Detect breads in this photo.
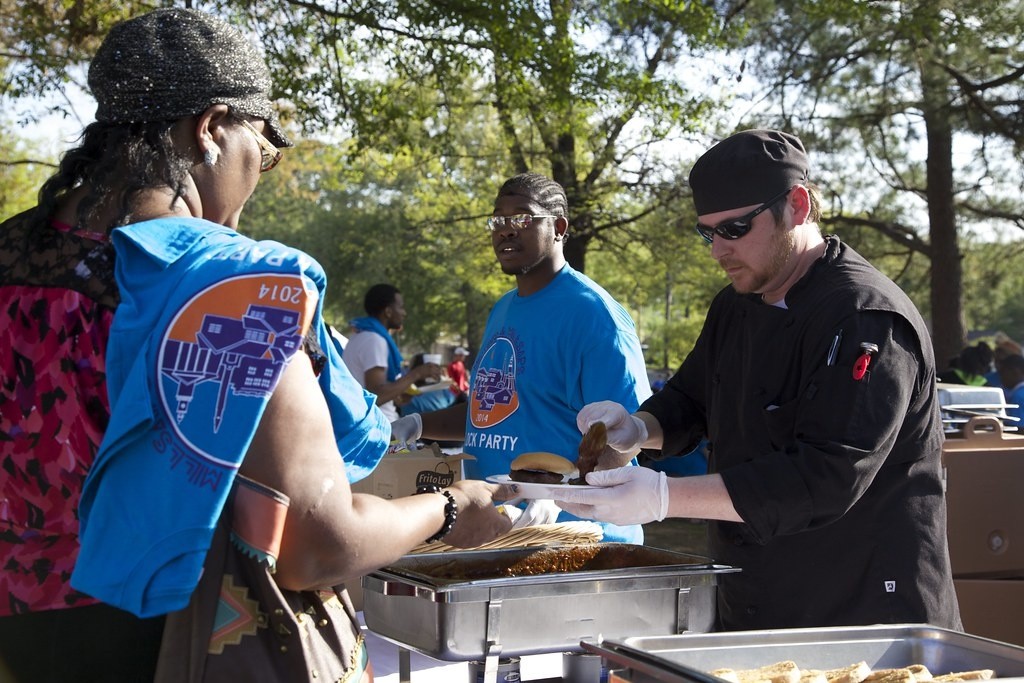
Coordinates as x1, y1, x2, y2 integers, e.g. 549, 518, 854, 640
708, 660, 998, 683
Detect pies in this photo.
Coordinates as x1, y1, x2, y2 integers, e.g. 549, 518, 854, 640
411, 520, 603, 553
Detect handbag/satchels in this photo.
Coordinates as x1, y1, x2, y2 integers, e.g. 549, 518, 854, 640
151, 471, 374, 683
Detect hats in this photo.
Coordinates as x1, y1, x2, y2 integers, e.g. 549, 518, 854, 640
455, 348, 469, 356
87, 7, 293, 148
688, 129, 810, 216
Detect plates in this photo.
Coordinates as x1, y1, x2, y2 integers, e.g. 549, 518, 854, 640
485, 474, 606, 499
418, 378, 454, 394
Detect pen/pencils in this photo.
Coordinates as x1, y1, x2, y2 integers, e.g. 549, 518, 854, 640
827, 329, 843, 366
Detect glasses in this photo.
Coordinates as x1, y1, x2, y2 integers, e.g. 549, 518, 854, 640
696, 187, 810, 243
195, 107, 283, 172
486, 215, 558, 231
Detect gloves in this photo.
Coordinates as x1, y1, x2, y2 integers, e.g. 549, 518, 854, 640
576, 400, 649, 453
554, 466, 669, 526
390, 412, 423, 446
503, 498, 562, 533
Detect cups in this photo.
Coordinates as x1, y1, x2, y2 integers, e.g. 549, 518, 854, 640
423, 354, 441, 382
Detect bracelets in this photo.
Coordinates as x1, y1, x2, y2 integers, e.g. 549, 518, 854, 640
410, 486, 458, 545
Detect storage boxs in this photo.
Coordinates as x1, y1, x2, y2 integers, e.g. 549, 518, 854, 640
345, 442, 477, 610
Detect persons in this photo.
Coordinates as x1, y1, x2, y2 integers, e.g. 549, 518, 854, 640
555, 129, 970, 636
446, 347, 470, 400
389, 173, 658, 548
0, 7, 520, 683
338, 284, 442, 425
937, 340, 1024, 434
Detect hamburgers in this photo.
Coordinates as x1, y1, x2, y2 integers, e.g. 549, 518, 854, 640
509, 453, 575, 484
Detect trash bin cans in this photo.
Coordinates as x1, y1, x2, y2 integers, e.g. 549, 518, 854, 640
937, 383, 1007, 433
